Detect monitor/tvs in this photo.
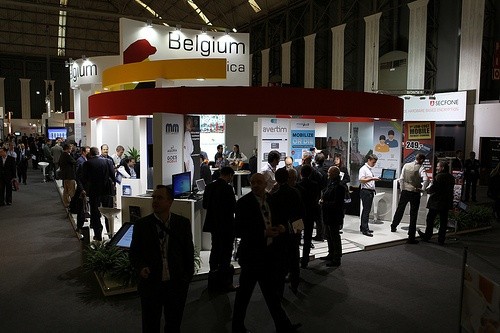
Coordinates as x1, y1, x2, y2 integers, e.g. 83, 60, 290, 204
382, 169, 396, 179
172, 171, 191, 197
47, 127, 68, 140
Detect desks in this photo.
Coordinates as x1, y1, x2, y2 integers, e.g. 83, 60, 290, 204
121, 193, 203, 256
233, 170, 251, 198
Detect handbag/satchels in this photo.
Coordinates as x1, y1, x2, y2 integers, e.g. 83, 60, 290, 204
69, 190, 82, 213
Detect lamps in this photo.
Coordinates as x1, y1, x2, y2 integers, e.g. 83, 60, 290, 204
147, 20, 207, 34
65, 54, 94, 67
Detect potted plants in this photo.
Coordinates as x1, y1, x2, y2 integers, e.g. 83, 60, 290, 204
81, 239, 131, 290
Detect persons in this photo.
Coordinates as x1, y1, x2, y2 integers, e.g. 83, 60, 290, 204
358, 155, 381, 237
0, 148, 17, 206
464, 152, 480, 202
201, 144, 351, 292
390, 153, 429, 245
59, 139, 140, 242
183, 117, 195, 194
232, 172, 302, 333
0, 133, 63, 185
452, 150, 465, 201
419, 161, 455, 243
129, 186, 194, 333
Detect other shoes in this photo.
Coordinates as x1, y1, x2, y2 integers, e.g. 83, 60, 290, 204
418, 230, 426, 241
326, 262, 340, 266
320, 256, 331, 260
366, 233, 373, 237
292, 322, 302, 332
93, 236, 102, 241
77, 233, 85, 240
312, 236, 324, 242
288, 285, 297, 293
368, 230, 373, 233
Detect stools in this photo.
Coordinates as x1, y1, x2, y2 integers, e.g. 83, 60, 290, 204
38, 162, 49, 183
98, 207, 120, 239
369, 192, 385, 224
